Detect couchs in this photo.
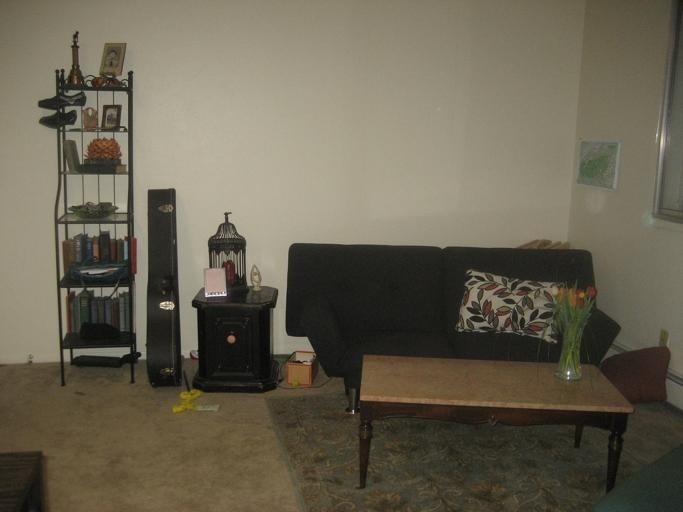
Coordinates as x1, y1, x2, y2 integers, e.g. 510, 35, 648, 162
283, 237, 621, 418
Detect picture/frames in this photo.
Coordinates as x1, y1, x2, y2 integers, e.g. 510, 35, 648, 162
97, 41, 127, 77
99, 103, 122, 132
573, 138, 622, 193
645, 0, 683, 228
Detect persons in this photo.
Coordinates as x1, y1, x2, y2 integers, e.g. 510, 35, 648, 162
105, 50, 118, 67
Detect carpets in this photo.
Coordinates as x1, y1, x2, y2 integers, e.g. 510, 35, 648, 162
259, 382, 682, 512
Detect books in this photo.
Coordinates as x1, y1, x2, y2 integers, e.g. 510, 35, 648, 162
63, 140, 80, 174
63, 232, 136, 333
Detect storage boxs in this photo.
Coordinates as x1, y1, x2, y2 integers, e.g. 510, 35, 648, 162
283, 349, 320, 387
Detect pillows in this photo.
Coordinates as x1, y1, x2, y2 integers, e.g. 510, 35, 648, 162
595, 343, 673, 405
454, 265, 574, 346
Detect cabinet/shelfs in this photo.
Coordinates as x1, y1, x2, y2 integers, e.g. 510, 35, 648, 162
185, 283, 284, 395
51, 66, 138, 389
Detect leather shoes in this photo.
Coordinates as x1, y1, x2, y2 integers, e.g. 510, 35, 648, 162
38, 91, 87, 109
38, 109, 77, 129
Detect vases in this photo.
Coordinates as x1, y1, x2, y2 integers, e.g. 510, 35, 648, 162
551, 307, 593, 382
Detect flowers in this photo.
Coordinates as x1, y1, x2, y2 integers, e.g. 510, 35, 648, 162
525, 276, 607, 385
